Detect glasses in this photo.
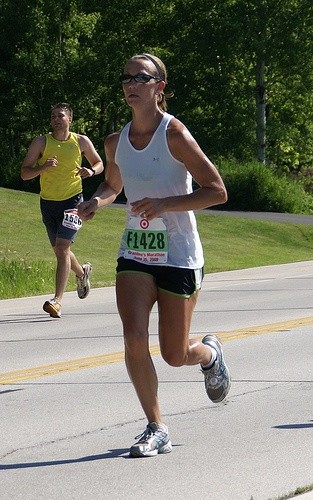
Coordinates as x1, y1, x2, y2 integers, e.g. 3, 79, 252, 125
119, 72, 161, 84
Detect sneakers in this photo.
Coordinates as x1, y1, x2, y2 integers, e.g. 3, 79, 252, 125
130, 422, 172, 457
76, 262, 92, 299
201, 334, 231, 403
43, 298, 62, 318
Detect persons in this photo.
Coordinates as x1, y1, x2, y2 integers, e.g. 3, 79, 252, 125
21, 102, 104, 318
76, 53, 233, 459
